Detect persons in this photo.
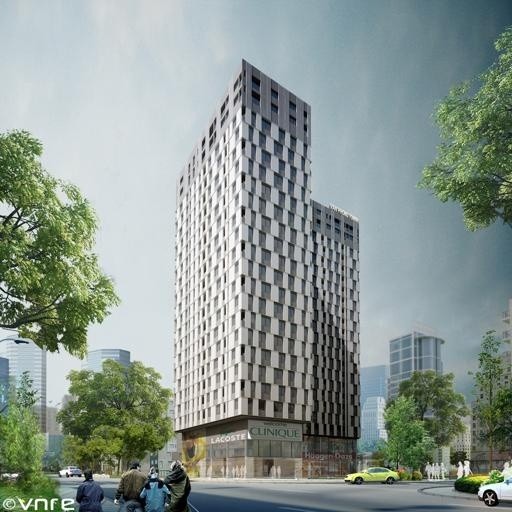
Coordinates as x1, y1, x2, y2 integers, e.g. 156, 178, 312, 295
114, 461, 147, 511
164, 459, 192, 512
140, 466, 171, 511
76, 469, 105, 511
